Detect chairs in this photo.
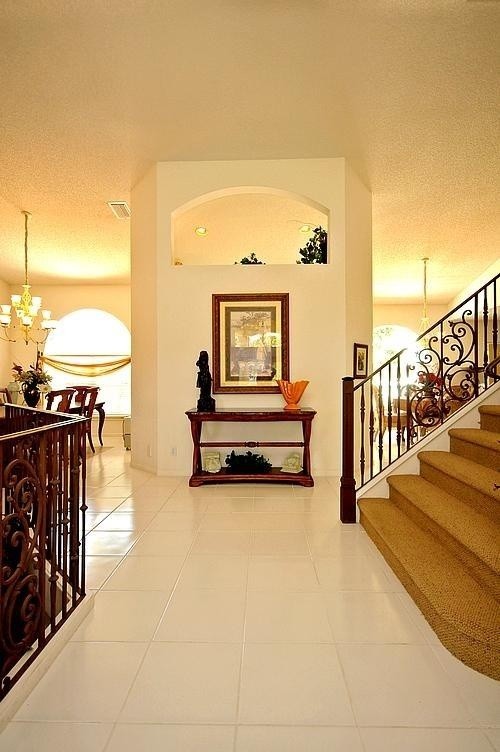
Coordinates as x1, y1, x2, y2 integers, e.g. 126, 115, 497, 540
0, 385, 99, 454
372, 385, 415, 447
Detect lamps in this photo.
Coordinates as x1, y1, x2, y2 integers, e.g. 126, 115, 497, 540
416, 257, 435, 347
0, 211, 59, 347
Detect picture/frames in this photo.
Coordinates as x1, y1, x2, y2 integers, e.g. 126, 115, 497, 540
354, 343, 368, 379
211, 292, 291, 395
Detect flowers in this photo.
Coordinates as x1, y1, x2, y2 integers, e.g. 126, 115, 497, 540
417, 370, 444, 389
8, 351, 53, 393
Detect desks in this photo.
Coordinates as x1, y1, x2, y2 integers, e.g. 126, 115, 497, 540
0, 401, 107, 447
392, 398, 430, 437
184, 407, 317, 488
